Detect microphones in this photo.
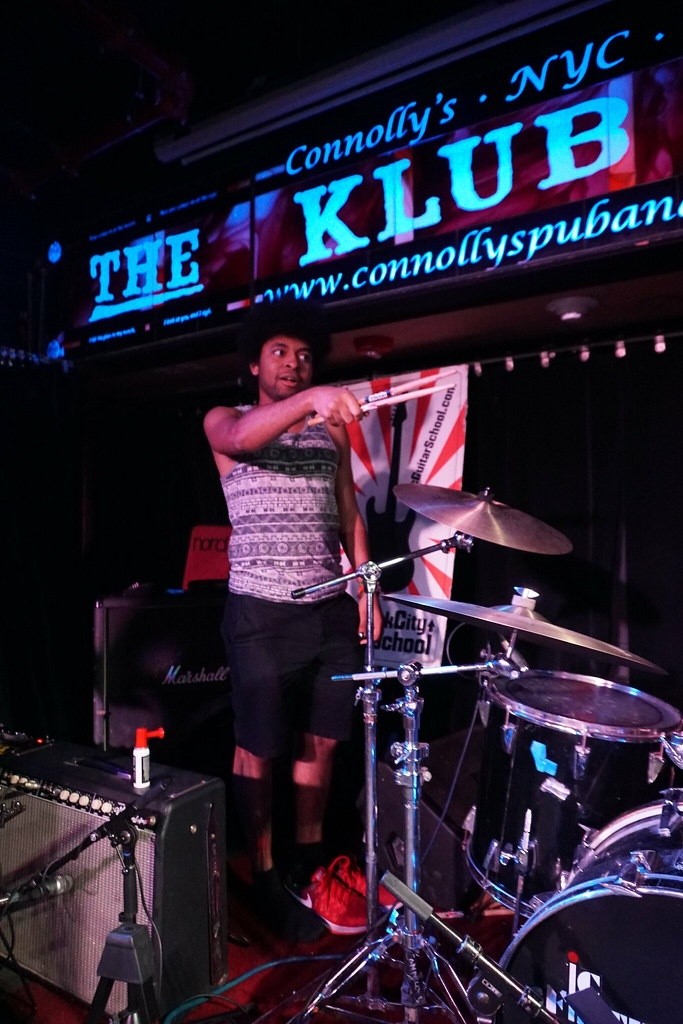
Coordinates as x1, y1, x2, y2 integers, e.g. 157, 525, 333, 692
0, 875, 72, 905
496, 628, 531, 671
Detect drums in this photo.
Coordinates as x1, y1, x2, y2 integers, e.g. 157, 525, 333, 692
461, 664, 683, 1024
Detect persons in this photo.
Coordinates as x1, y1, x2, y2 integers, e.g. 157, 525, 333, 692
202, 301, 387, 943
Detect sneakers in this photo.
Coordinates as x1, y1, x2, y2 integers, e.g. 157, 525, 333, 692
283, 864, 377, 935
327, 854, 403, 910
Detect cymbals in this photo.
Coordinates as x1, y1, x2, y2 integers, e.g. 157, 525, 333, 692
391, 481, 575, 558
380, 586, 666, 675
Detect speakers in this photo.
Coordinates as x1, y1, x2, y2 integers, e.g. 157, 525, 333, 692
0, 732, 228, 1024
355, 720, 514, 915
92, 598, 238, 760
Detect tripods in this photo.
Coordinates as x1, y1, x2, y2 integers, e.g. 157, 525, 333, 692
11, 779, 168, 1024
250, 542, 517, 1024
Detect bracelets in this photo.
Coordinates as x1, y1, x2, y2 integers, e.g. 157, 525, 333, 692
356, 580, 382, 599
